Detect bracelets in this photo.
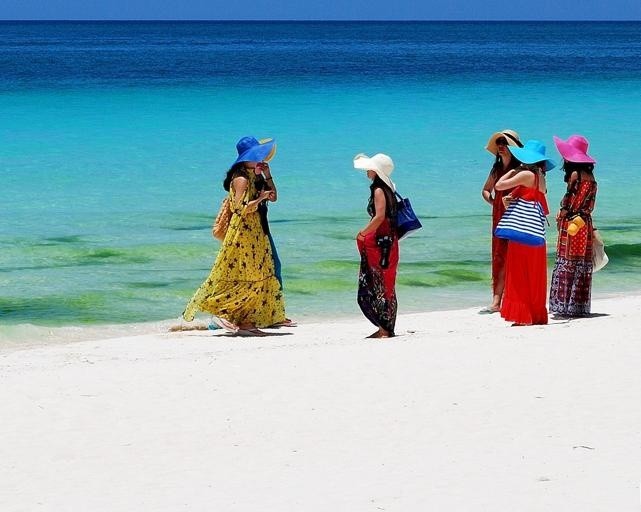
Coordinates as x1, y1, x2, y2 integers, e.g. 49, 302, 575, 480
359, 232, 365, 238
264, 177, 272, 181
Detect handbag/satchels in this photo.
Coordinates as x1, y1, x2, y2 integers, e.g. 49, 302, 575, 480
398, 197, 421, 240
494, 196, 545, 245
212, 197, 233, 241
591, 228, 609, 274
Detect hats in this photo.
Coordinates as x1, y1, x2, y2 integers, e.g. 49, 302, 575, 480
484, 129, 524, 157
553, 135, 597, 164
507, 140, 557, 171
229, 137, 276, 168
354, 153, 396, 194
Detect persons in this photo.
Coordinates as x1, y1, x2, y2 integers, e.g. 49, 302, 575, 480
477, 129, 525, 314
255, 138, 291, 325
179, 135, 287, 336
548, 134, 597, 319
494, 140, 556, 325
352, 153, 399, 337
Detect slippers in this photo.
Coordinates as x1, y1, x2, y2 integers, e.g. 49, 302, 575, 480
268, 318, 298, 327
213, 316, 236, 332
366, 331, 388, 337
238, 327, 268, 336
478, 307, 501, 314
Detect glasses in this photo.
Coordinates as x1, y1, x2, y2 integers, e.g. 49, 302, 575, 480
495, 139, 508, 146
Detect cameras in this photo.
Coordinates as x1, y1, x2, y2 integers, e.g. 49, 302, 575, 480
376, 233, 394, 269
